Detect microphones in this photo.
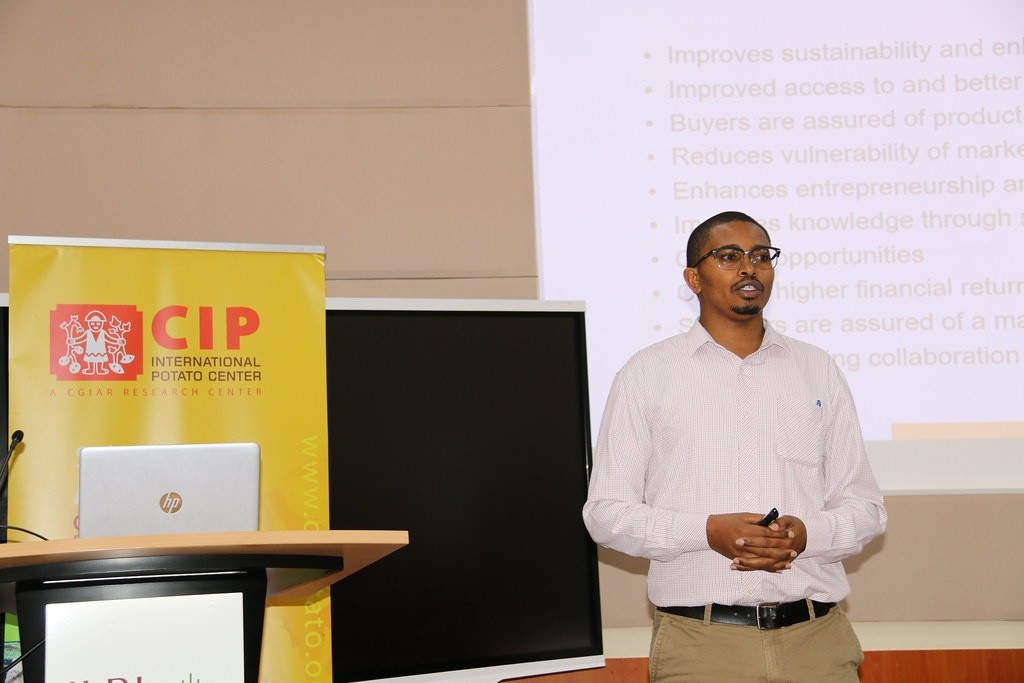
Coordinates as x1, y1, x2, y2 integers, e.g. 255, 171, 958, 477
1, 430, 23, 481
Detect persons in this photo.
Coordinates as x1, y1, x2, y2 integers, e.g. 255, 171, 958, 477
583, 210, 888, 683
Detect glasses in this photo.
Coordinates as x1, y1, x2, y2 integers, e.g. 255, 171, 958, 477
692, 246, 780, 271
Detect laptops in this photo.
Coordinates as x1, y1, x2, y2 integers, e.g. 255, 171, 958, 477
75, 443, 261, 539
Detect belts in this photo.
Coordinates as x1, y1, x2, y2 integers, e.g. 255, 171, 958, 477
656, 598, 837, 631
